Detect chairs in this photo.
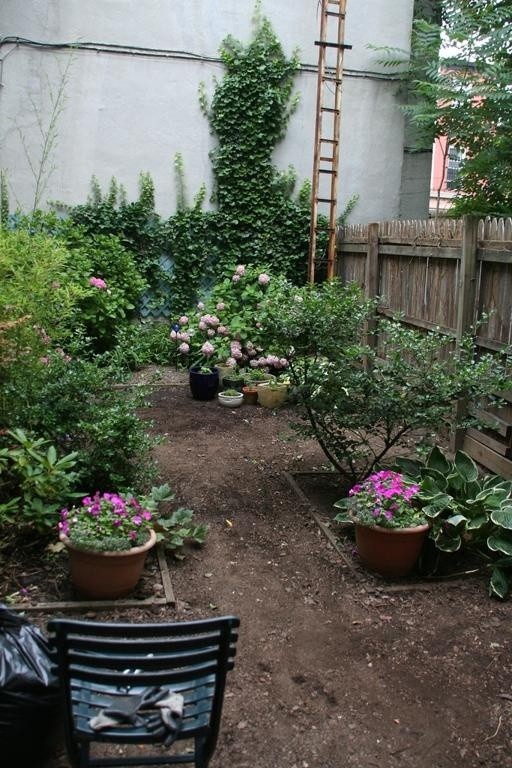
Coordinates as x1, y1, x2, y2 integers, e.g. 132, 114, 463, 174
45, 615, 241, 768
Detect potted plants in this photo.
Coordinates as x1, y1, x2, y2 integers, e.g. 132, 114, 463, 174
189, 366, 287, 408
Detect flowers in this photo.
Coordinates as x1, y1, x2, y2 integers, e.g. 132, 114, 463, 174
170, 263, 306, 374
57, 489, 153, 552
348, 470, 428, 528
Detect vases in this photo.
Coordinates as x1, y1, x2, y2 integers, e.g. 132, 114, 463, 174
59, 528, 157, 600
349, 509, 430, 579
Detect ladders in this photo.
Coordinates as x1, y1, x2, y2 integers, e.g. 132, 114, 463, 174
308, 0, 352, 285
